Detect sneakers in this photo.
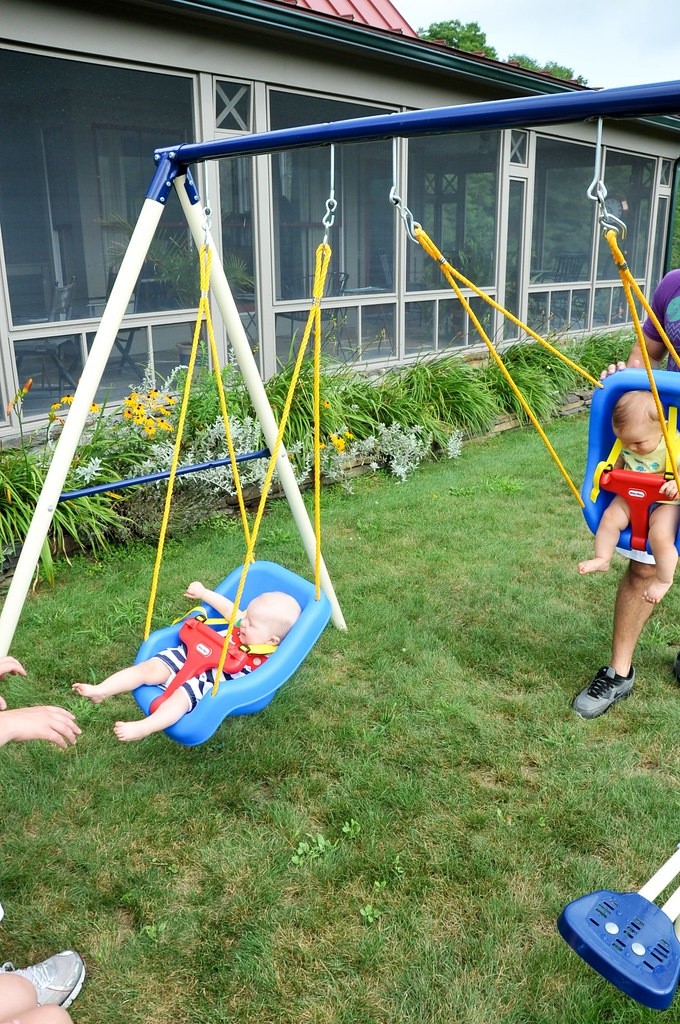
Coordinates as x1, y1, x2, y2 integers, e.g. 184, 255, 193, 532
2, 947, 88, 1013
572, 663, 637, 720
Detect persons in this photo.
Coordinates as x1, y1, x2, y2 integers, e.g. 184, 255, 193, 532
569, 268, 680, 721
578, 389, 680, 604
71, 581, 302, 741
0, 972, 74, 1024
0, 655, 88, 1010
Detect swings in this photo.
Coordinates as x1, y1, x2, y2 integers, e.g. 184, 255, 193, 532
132, 242, 332, 744
415, 229, 680, 555
